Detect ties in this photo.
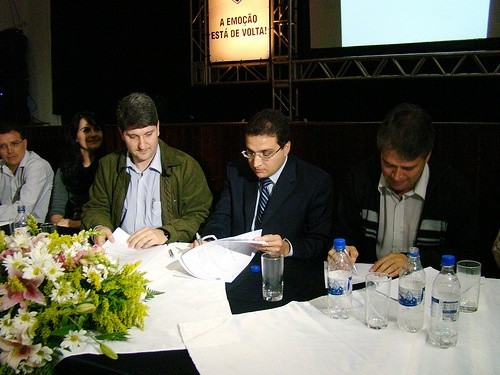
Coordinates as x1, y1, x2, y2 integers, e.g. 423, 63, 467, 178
254, 177, 272, 229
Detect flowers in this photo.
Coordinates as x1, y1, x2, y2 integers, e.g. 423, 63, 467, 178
0, 212, 164, 375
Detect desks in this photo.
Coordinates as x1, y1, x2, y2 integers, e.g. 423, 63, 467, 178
53, 251, 500, 375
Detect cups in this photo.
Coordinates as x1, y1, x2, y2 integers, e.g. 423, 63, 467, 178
365, 272, 392, 330
456, 260, 482, 314
9, 218, 15, 234
261, 251, 285, 302
40, 222, 57, 234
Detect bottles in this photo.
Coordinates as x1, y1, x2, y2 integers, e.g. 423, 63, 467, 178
426, 255, 461, 349
13, 204, 31, 236
396, 246, 426, 333
328, 238, 352, 320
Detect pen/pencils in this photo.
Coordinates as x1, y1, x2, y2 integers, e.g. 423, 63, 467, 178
196, 233, 202, 244
169, 249, 174, 256
344, 249, 360, 275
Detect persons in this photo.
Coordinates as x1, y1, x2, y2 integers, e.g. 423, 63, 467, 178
48, 110, 118, 228
81, 91, 212, 249
191, 110, 334, 261
326, 102, 481, 278
0, 123, 54, 236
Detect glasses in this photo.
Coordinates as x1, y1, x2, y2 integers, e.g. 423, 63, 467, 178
242, 146, 283, 161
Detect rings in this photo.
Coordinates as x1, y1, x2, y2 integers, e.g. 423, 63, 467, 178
265, 246, 268, 251
